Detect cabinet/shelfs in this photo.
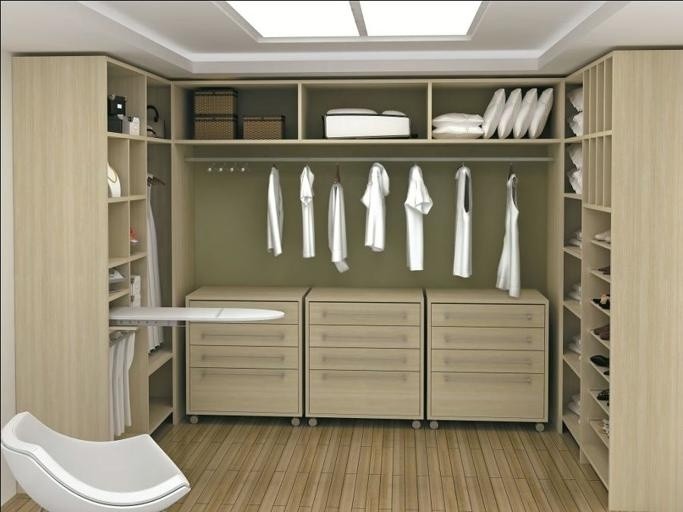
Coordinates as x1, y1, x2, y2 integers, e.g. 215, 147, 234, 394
172, 77, 565, 435
186, 287, 310, 425
11, 56, 172, 439
304, 287, 426, 429
426, 289, 550, 431
562, 50, 682, 511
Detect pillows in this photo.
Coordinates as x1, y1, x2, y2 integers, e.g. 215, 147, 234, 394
484, 88, 505, 140
432, 127, 483, 139
432, 113, 484, 126
529, 88, 554, 138
513, 88, 537, 138
499, 88, 521, 139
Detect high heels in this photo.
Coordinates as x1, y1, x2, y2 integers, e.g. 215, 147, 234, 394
593, 294, 610, 309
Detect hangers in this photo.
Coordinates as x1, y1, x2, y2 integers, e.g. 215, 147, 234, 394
148, 159, 518, 186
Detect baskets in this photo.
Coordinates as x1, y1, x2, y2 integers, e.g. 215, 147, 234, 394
193, 88, 284, 139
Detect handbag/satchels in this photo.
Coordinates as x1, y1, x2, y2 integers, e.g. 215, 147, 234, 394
147, 105, 165, 137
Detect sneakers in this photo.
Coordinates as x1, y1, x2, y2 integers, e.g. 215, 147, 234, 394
597, 389, 609, 406
598, 264, 610, 275
594, 324, 610, 340
595, 228, 610, 244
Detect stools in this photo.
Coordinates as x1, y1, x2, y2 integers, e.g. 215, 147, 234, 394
0, 412, 190, 510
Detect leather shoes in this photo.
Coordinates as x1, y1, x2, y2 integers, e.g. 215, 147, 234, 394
590, 354, 609, 375
